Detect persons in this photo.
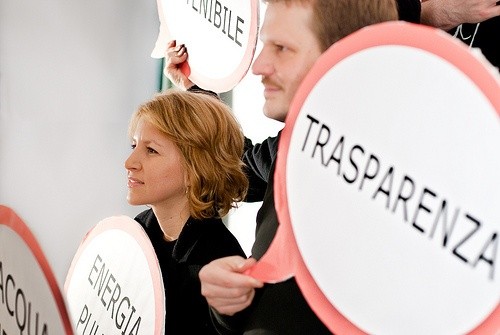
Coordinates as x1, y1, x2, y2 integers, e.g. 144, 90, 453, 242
163, 0, 398, 262
124, 88, 249, 335
419, 0, 500, 75
198, 255, 334, 335
